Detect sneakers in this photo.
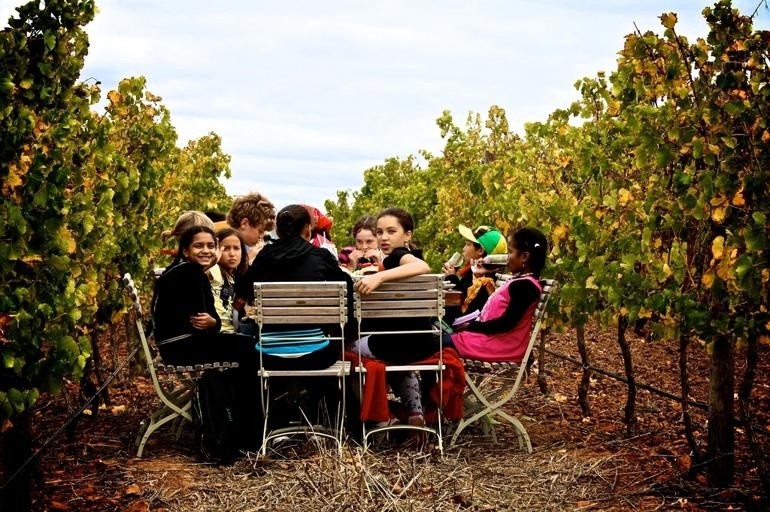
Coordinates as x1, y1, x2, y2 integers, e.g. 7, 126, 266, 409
376, 413, 427, 450
273, 434, 322, 450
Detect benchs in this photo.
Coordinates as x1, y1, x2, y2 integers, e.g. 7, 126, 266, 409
447, 272, 555, 455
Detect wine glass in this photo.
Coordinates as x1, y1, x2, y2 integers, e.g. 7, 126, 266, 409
470, 254, 508, 270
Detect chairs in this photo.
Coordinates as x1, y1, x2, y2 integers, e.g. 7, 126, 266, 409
254, 280, 352, 458
124, 269, 239, 457
353, 275, 448, 456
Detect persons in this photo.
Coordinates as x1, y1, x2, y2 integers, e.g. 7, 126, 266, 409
418, 224, 548, 451
149, 192, 432, 465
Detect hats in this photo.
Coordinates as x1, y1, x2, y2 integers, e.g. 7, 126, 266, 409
458, 224, 507, 254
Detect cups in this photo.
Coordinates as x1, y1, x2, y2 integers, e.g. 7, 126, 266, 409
446, 251, 464, 267
